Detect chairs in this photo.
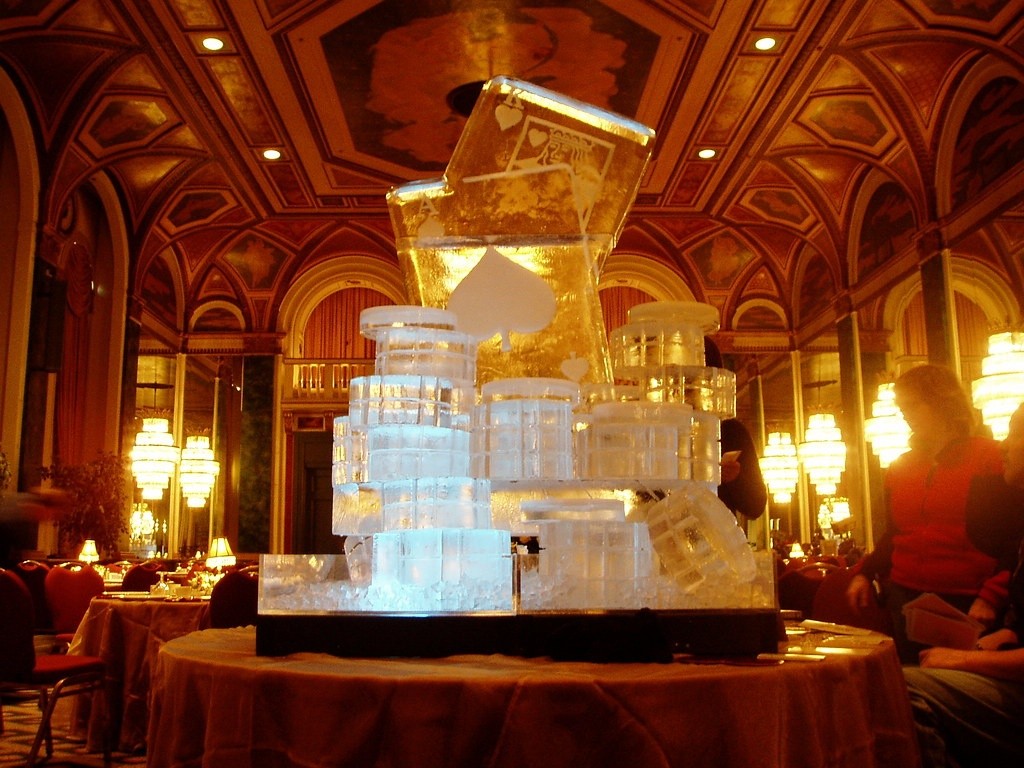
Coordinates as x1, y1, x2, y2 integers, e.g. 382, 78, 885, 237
771, 540, 892, 637
41, 560, 107, 655
10, 552, 259, 630
0, 568, 118, 768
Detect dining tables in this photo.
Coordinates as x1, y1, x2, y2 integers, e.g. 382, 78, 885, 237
144, 623, 924, 768
66, 591, 214, 758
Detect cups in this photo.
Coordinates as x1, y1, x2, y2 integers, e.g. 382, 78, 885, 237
110, 573, 122, 580
181, 568, 187, 572
176, 586, 191, 597
167, 584, 181, 597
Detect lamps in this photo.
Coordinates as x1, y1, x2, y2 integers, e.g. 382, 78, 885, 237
798, 402, 848, 496
205, 536, 236, 582
759, 419, 801, 504
865, 369, 917, 470
78, 540, 100, 567
179, 424, 220, 508
128, 406, 181, 500
972, 313, 1024, 442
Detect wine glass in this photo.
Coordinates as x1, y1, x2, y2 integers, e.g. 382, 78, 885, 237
153, 571, 170, 596
177, 563, 181, 572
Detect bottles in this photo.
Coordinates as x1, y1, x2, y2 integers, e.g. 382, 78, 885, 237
121, 565, 126, 579
106, 568, 110, 580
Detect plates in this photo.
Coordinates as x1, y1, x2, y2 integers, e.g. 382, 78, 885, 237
104, 591, 211, 601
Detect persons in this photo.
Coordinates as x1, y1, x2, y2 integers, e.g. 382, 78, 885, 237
511, 536, 540, 555
845, 363, 1018, 668
898, 401, 1024, 768
634, 334, 767, 521
